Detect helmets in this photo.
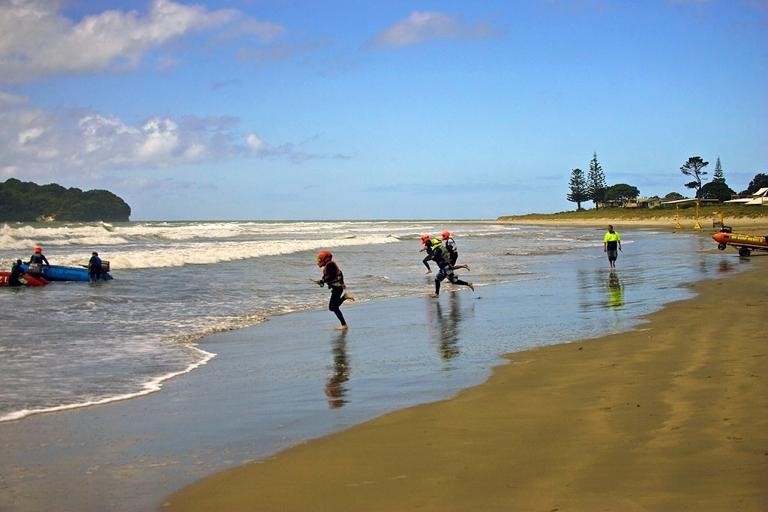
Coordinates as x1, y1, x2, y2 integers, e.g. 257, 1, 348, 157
35, 247, 42, 253
421, 234, 429, 243
316, 251, 333, 267
431, 238, 442, 251
442, 231, 450, 240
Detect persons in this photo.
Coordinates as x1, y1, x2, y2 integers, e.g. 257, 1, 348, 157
305, 250, 357, 333
418, 234, 436, 274
441, 231, 459, 283
430, 238, 475, 298
7, 259, 28, 288
21, 245, 50, 274
87, 251, 104, 286
602, 222, 624, 269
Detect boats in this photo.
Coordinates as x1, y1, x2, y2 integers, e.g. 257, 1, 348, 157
712, 225, 768, 250
12, 260, 114, 282
0, 272, 49, 287
